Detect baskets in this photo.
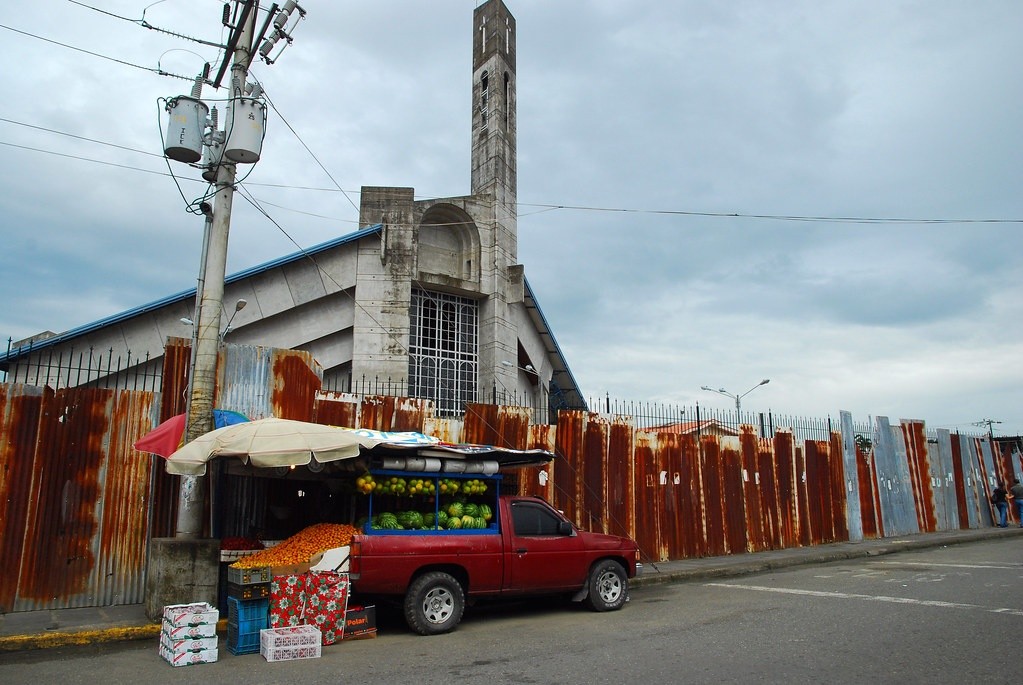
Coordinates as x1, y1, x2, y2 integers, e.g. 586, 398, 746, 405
259, 624, 321, 663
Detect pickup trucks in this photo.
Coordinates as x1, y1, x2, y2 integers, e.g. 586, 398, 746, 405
349, 494, 643, 635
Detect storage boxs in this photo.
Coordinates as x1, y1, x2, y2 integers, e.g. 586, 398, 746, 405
159, 601, 219, 667
220, 538, 377, 662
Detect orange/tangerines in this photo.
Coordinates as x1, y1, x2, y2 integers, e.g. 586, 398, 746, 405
357, 475, 488, 495
229, 522, 355, 567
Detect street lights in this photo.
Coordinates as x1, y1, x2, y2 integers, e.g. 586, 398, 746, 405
180, 299, 247, 337
701, 379, 770, 423
502, 361, 543, 393
174, 199, 231, 535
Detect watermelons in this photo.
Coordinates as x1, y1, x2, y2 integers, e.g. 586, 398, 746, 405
361, 495, 492, 535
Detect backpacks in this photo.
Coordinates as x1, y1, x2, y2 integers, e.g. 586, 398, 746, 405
990, 489, 998, 504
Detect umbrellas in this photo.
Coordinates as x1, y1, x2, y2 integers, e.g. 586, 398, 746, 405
131, 409, 253, 539
166, 414, 387, 477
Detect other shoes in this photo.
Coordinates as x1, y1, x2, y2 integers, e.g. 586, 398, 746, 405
1000, 524, 1007, 528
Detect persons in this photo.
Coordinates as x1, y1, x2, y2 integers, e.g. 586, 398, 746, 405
1009, 479, 1023, 528
993, 479, 1014, 528
